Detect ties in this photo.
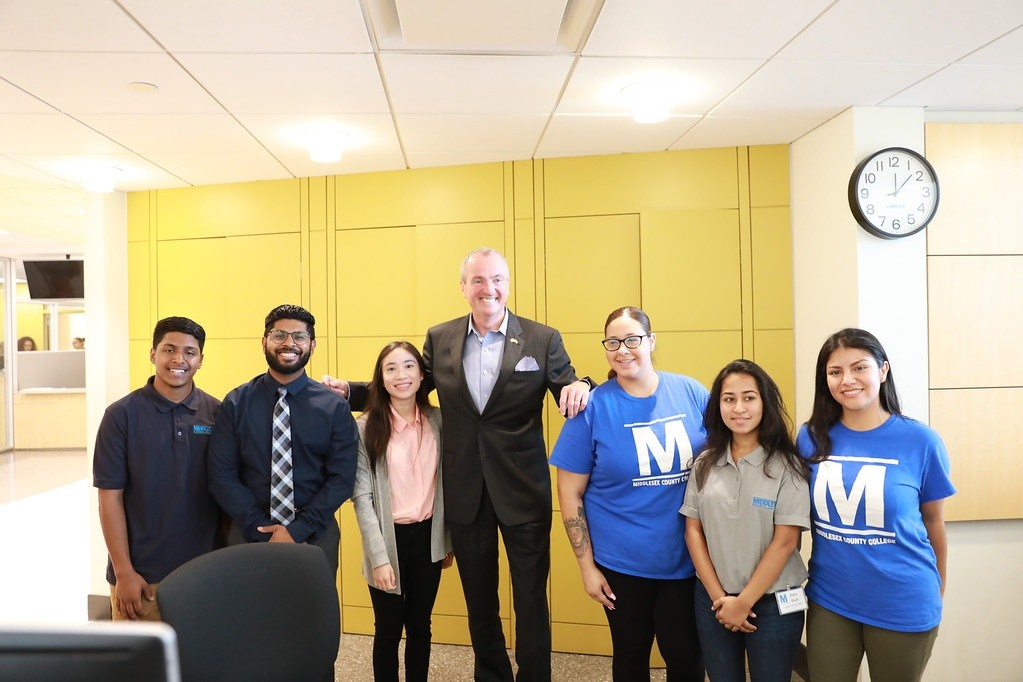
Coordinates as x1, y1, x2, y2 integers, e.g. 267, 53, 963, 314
270, 386, 295, 527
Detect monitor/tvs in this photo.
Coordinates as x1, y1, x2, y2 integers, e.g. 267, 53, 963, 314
23, 260, 84, 299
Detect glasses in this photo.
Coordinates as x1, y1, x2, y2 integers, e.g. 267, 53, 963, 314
265, 329, 311, 346
602, 334, 649, 352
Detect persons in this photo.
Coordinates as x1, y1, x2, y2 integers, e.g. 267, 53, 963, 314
797, 327, 957, 682
678, 359, 810, 682
206, 304, 359, 574
351, 341, 455, 682
547, 306, 711, 682
93, 316, 224, 625
18, 336, 36, 351
72, 337, 85, 349
321, 248, 599, 682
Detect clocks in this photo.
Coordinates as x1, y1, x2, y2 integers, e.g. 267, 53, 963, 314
847, 146, 941, 239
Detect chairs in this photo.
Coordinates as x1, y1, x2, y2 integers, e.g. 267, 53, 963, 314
156, 542, 341, 682
0, 622, 183, 682
